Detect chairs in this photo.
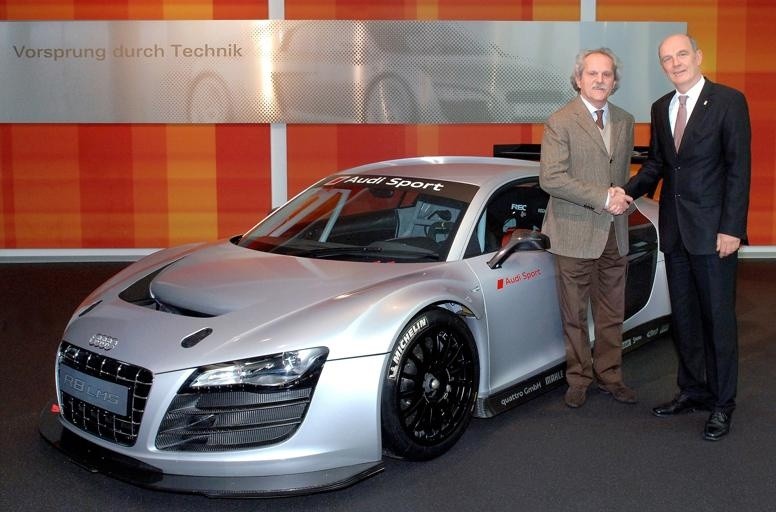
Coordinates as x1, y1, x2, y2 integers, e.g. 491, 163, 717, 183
409, 187, 543, 250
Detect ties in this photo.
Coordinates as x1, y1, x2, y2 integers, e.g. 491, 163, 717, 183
595, 109, 605, 130
672, 95, 690, 153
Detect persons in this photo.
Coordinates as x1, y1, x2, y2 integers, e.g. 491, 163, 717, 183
607, 34, 752, 440
540, 46, 640, 406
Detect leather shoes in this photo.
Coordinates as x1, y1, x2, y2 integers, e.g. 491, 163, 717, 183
597, 377, 641, 404
565, 382, 588, 409
650, 397, 695, 419
703, 408, 731, 442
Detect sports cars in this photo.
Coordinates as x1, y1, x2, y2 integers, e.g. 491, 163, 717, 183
170, 20, 578, 124
34, 141, 677, 498
